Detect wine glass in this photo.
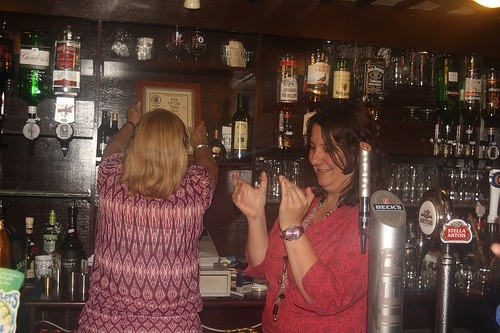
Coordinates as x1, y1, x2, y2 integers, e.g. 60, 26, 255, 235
167, 31, 187, 64
187, 32, 208, 64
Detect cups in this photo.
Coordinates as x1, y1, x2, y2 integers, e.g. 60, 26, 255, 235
34, 256, 86, 302
405, 266, 439, 291
455, 266, 491, 296
442, 167, 488, 205
254, 158, 303, 200
383, 162, 438, 207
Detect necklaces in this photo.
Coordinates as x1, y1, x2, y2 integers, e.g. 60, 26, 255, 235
303, 196, 342, 229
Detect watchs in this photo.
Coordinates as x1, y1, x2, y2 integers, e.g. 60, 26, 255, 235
280, 224, 304, 240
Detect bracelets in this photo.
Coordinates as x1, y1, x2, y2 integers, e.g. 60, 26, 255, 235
194, 144, 211, 150
123, 121, 136, 128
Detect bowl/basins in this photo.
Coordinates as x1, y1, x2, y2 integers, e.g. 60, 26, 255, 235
226, 170, 252, 193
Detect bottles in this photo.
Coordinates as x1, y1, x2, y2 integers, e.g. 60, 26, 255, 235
438, 54, 500, 158
96, 110, 119, 167
17, 217, 41, 286
219, 39, 249, 69
43, 210, 63, 278
111, 35, 155, 62
276, 42, 392, 158
20, 29, 50, 153
62, 207, 88, 280
53, 30, 80, 156
0, 220, 11, 268
208, 126, 226, 161
231, 94, 250, 162
390, 48, 438, 86
0, 20, 15, 135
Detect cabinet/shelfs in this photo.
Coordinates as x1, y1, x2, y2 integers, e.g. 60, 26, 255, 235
0, 10, 500, 268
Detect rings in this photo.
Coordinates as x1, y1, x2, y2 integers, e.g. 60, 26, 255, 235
205, 132, 209, 136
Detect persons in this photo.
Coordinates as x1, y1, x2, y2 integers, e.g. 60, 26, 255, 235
232, 101, 376, 333
78, 102, 218, 333
